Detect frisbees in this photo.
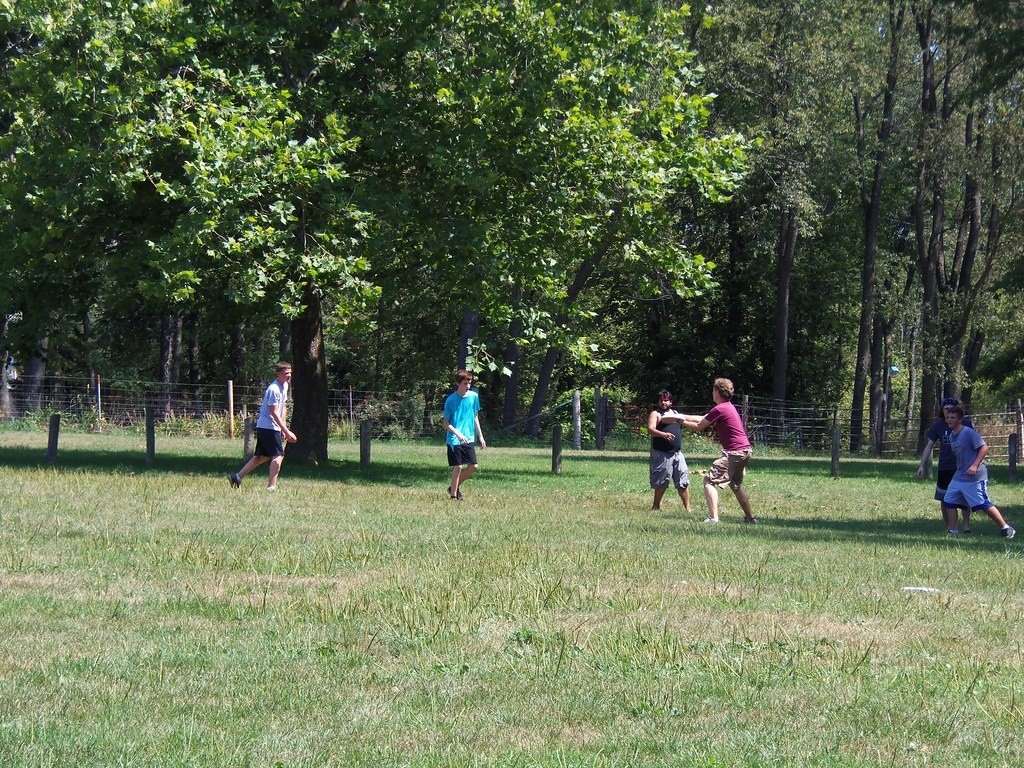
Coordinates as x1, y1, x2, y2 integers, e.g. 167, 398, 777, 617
661, 416, 686, 423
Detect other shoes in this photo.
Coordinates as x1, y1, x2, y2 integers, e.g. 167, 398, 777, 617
703, 516, 716, 523
965, 529, 971, 533
744, 516, 757, 523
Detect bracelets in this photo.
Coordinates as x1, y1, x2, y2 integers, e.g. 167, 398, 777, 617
676, 419, 681, 423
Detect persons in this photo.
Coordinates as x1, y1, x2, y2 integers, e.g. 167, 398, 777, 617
664, 378, 757, 526
647, 391, 701, 514
918, 399, 976, 535
5, 356, 17, 393
443, 370, 486, 501
941, 406, 1016, 540
228, 362, 297, 493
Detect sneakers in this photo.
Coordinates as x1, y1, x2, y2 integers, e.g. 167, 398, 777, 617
228, 475, 241, 487
447, 486, 463, 501
1000, 525, 1015, 539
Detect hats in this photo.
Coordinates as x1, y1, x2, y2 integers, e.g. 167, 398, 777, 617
939, 399, 958, 417
